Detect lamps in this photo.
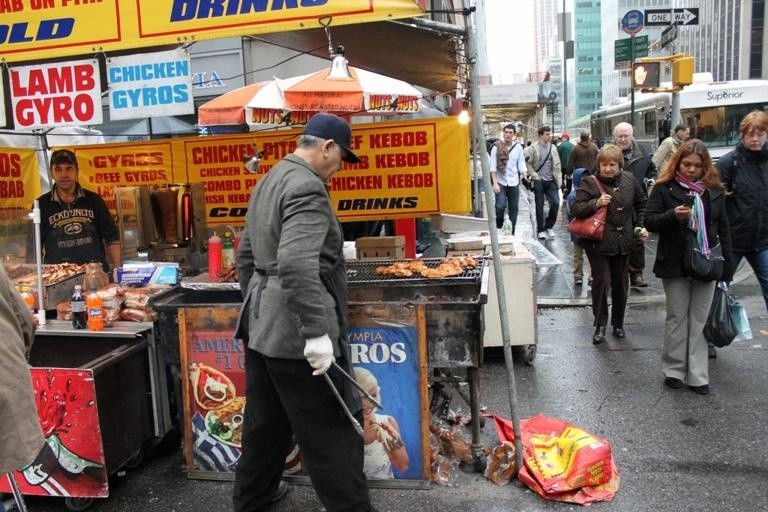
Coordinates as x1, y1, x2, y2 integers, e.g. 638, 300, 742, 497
456, 84, 471, 126
323, 20, 355, 83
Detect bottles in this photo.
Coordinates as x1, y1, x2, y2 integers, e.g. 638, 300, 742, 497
113, 268, 118, 282
71, 286, 87, 329
208, 231, 222, 280
90, 275, 97, 288
22, 287, 35, 315
222, 232, 236, 270
108, 264, 113, 283
14, 286, 22, 297
16, 433, 108, 498
117, 267, 123, 282
137, 247, 149, 261
87, 288, 104, 331
234, 228, 244, 257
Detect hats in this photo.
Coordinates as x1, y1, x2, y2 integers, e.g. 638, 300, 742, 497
51, 150, 78, 164
580, 127, 589, 139
299, 113, 362, 164
559, 132, 569, 140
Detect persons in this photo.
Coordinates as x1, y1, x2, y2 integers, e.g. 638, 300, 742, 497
351, 367, 410, 482
524, 126, 563, 240
557, 122, 692, 344
25, 150, 122, 283
232, 112, 378, 512
489, 123, 531, 235
644, 138, 733, 394
708, 110, 768, 359
0, 262, 45, 482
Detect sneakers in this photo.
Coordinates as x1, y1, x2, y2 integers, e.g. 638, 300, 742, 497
630, 272, 648, 287
538, 228, 546, 239
233, 479, 290, 512
545, 224, 555, 238
574, 276, 583, 284
707, 344, 717, 358
563, 188, 568, 200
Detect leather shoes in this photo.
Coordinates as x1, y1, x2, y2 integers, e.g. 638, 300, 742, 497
613, 320, 625, 338
665, 376, 682, 389
593, 326, 606, 344
689, 383, 709, 395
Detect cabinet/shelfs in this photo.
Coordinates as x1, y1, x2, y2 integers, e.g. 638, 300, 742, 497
0, 321, 170, 508
445, 230, 540, 364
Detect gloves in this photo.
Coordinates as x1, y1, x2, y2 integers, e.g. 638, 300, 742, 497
304, 333, 336, 375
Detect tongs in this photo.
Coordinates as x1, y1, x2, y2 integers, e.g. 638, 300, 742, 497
324, 363, 383, 437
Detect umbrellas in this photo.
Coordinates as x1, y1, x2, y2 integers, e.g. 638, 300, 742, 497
197, 64, 423, 131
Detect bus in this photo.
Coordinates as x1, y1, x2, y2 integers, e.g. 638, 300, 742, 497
586, 79, 768, 160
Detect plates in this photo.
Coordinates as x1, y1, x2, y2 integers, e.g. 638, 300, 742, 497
195, 364, 235, 410
204, 396, 248, 448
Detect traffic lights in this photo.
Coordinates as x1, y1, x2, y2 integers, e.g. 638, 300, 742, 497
632, 62, 660, 87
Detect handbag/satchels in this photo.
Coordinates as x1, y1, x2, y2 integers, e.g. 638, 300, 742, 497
522, 174, 535, 192
683, 232, 725, 281
567, 205, 608, 240
727, 293, 755, 343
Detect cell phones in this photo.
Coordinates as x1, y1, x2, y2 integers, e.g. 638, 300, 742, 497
682, 193, 696, 210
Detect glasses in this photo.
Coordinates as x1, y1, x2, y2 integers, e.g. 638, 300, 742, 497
504, 131, 514, 134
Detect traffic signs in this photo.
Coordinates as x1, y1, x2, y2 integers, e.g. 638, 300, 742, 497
634, 35, 649, 58
644, 10, 671, 26
614, 38, 631, 71
673, 8, 700, 26
660, 22, 677, 49
